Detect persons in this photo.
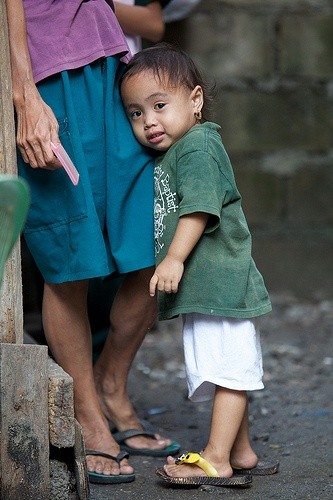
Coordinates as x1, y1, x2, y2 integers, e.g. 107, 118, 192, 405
115, 0, 203, 76
6, 0, 182, 483
120, 43, 280, 486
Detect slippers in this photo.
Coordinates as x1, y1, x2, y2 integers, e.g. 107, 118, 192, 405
156, 451, 252, 485
85, 440, 136, 482
230, 449, 279, 476
112, 416, 181, 457
